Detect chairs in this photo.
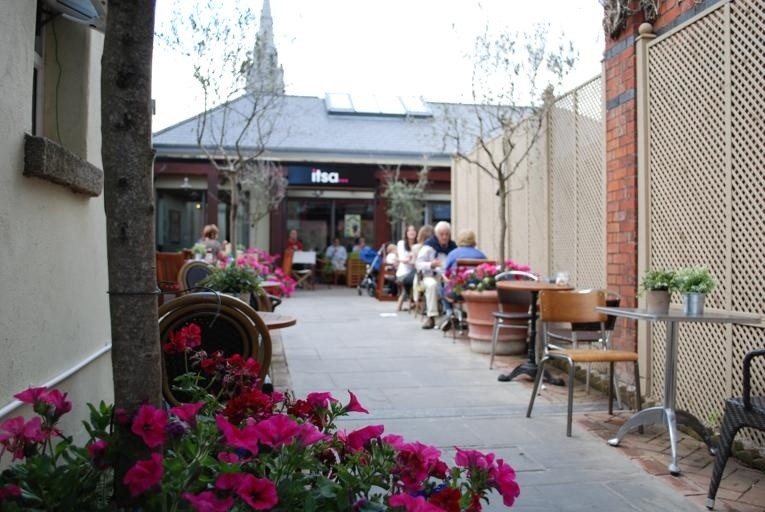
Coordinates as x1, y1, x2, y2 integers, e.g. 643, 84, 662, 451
178, 260, 214, 293
706, 349, 765, 511
158, 293, 272, 407
537, 289, 623, 409
445, 260, 495, 344
489, 270, 538, 369
283, 250, 426, 319
157, 250, 185, 304
184, 248, 213, 260
526, 291, 644, 436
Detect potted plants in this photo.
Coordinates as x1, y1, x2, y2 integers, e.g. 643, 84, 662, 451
670, 266, 714, 314
636, 270, 676, 313
194, 263, 265, 306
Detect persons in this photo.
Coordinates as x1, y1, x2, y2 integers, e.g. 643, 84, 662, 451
284, 228, 304, 274
193, 224, 232, 259
325, 237, 348, 269
351, 221, 488, 331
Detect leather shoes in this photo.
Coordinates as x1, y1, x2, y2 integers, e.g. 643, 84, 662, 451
421, 317, 434, 329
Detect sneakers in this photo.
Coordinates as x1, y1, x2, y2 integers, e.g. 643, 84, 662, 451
441, 316, 455, 331
